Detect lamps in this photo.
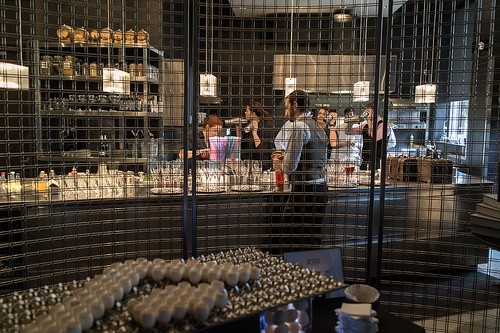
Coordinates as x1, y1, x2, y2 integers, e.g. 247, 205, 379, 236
285, 0, 296, 98
414, 0, 438, 103
0, 0, 30, 90
102, 0, 130, 95
353, 0, 370, 102
333, 0, 355, 24
200, 0, 217, 98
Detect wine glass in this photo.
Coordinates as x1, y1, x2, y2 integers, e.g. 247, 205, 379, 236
324, 158, 390, 187
146, 160, 264, 194
127, 126, 155, 140
68, 94, 121, 112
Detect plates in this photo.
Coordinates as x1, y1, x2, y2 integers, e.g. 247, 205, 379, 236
61, 149, 91, 158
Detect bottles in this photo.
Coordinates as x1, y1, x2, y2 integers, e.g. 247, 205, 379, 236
360, 111, 369, 121
1, 170, 144, 197
275, 155, 284, 192
121, 95, 164, 112
103, 134, 109, 157
98, 131, 105, 157
410, 133, 414, 146
244, 121, 259, 133
323, 115, 334, 123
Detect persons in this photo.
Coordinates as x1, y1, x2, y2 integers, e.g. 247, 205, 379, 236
179, 114, 223, 160
271, 90, 330, 244
316, 101, 397, 171
235, 101, 274, 170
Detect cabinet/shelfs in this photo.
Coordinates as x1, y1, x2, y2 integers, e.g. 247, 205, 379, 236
30, 40, 164, 173
387, 99, 430, 158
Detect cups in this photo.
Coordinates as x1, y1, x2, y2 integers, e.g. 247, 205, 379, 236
224, 118, 248, 124
344, 117, 359, 123
